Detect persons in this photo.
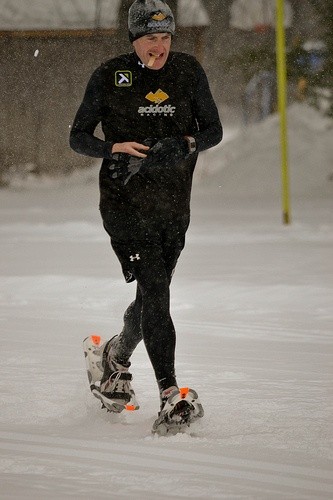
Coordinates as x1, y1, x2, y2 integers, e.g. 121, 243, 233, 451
69, 0, 224, 436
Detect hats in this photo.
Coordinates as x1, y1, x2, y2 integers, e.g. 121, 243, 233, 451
127, 0, 175, 44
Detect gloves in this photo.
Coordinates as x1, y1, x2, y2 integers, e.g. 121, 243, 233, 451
142, 138, 187, 175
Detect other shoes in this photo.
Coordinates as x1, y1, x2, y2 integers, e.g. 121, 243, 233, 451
99, 333, 131, 414
157, 392, 198, 425
